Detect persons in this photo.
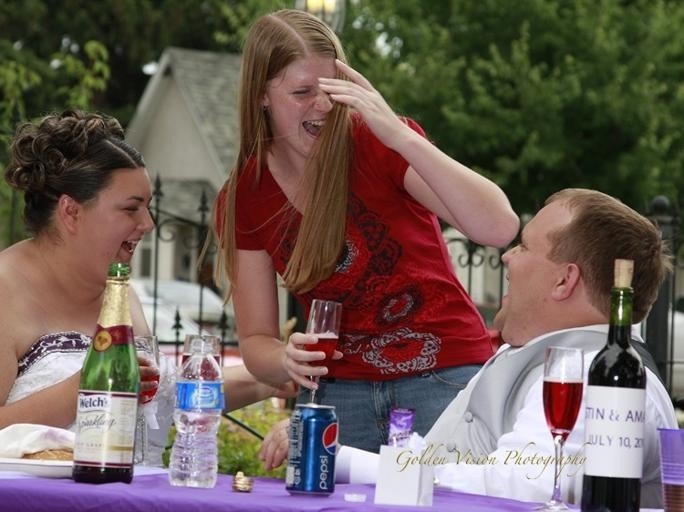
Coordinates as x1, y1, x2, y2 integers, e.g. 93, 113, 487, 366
211, 7, 522, 453
258, 187, 680, 506
0, 109, 300, 461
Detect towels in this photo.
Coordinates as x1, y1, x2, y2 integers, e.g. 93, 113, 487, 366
0, 423, 76, 459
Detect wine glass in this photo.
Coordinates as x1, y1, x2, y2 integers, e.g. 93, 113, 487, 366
135, 331, 160, 470
300, 298, 344, 407
537, 344, 586, 512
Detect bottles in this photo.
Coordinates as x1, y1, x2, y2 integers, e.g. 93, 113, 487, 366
576, 258, 649, 512
165, 337, 225, 490
70, 260, 141, 485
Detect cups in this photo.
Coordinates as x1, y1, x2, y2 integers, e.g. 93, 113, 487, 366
655, 426, 684, 512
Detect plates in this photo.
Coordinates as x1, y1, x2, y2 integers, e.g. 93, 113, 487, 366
0, 457, 75, 479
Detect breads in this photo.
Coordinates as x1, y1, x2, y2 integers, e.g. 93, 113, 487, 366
23, 451, 74, 460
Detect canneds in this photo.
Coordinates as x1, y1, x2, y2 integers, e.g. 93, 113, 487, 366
280, 402, 339, 496
